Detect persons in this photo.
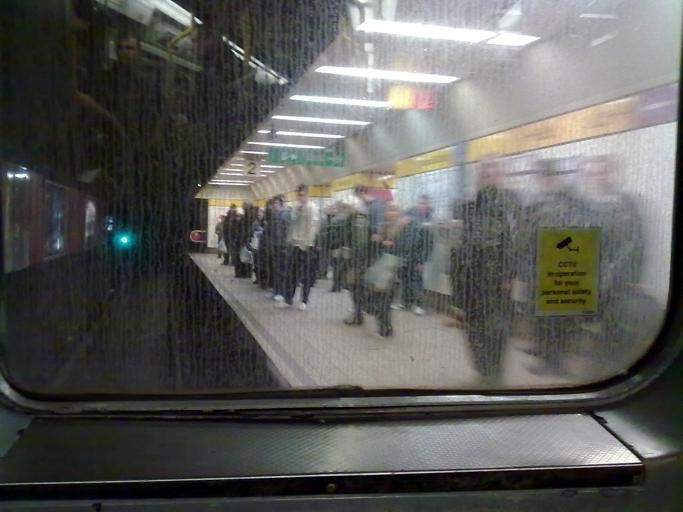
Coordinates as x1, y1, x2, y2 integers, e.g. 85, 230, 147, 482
214, 184, 434, 338
0, 0, 161, 138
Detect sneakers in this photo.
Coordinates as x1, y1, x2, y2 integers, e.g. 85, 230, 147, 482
265, 291, 307, 311
413, 304, 425, 315
390, 304, 406, 310
